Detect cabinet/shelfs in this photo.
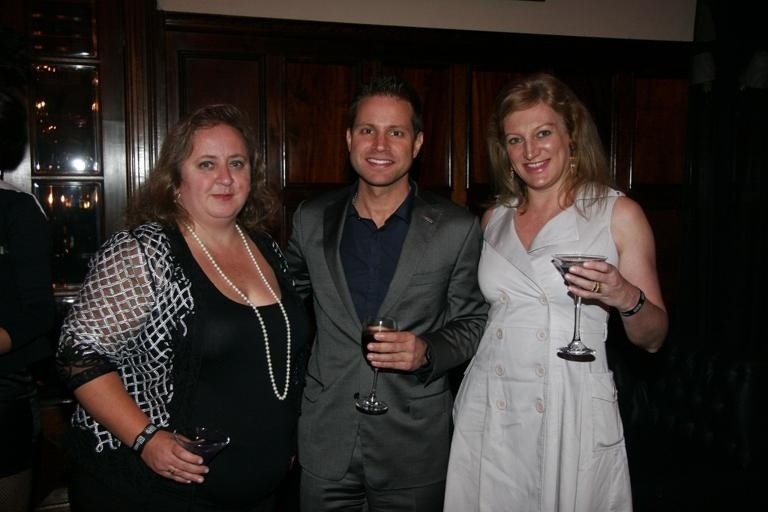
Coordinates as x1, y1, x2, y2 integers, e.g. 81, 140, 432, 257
373, 24, 615, 209
614, 41, 710, 382
152, 12, 367, 252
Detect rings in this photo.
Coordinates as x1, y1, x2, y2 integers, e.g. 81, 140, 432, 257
168, 467, 175, 474
594, 281, 600, 292
591, 281, 597, 292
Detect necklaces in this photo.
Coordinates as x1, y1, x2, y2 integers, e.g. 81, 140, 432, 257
181, 220, 291, 401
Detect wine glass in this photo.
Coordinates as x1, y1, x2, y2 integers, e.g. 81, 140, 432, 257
353, 315, 397, 414
549, 253, 609, 357
170, 424, 231, 498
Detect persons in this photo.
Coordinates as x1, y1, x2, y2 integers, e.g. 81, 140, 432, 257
441, 73, 669, 512
54, 103, 318, 512
284, 70, 489, 512
0, 189, 53, 512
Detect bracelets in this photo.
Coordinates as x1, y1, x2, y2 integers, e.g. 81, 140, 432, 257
621, 289, 644, 317
131, 423, 160, 456
